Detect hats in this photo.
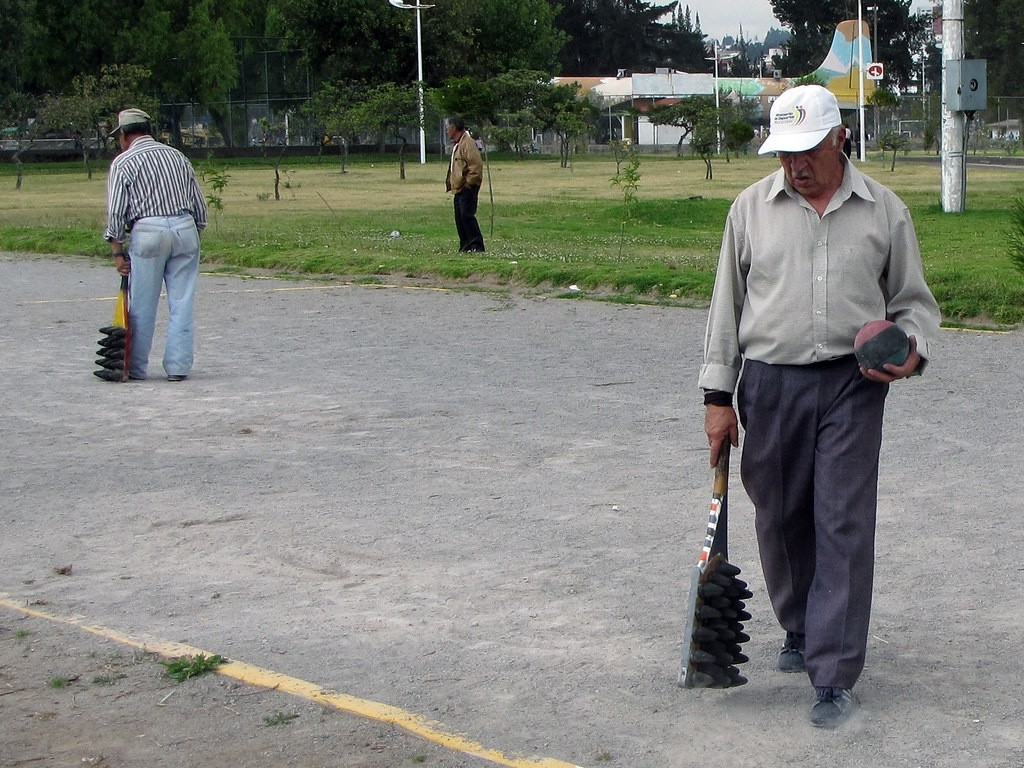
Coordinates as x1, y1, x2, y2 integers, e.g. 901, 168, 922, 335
757, 84, 841, 154
108, 108, 151, 136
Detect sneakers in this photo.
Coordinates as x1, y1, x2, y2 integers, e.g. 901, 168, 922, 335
780, 641, 809, 672
808, 684, 853, 728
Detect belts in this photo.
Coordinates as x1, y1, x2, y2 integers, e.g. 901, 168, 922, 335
128, 217, 140, 230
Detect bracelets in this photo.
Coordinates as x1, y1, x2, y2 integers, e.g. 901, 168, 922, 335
113, 253, 125, 258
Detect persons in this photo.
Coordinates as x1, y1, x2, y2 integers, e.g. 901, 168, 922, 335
841, 123, 861, 159
106, 108, 209, 381
698, 86, 940, 727
445, 117, 485, 252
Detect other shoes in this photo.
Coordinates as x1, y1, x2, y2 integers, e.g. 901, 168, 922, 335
167, 374, 187, 380
128, 374, 145, 380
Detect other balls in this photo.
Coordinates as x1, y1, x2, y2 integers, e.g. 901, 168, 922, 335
853, 318, 910, 372
